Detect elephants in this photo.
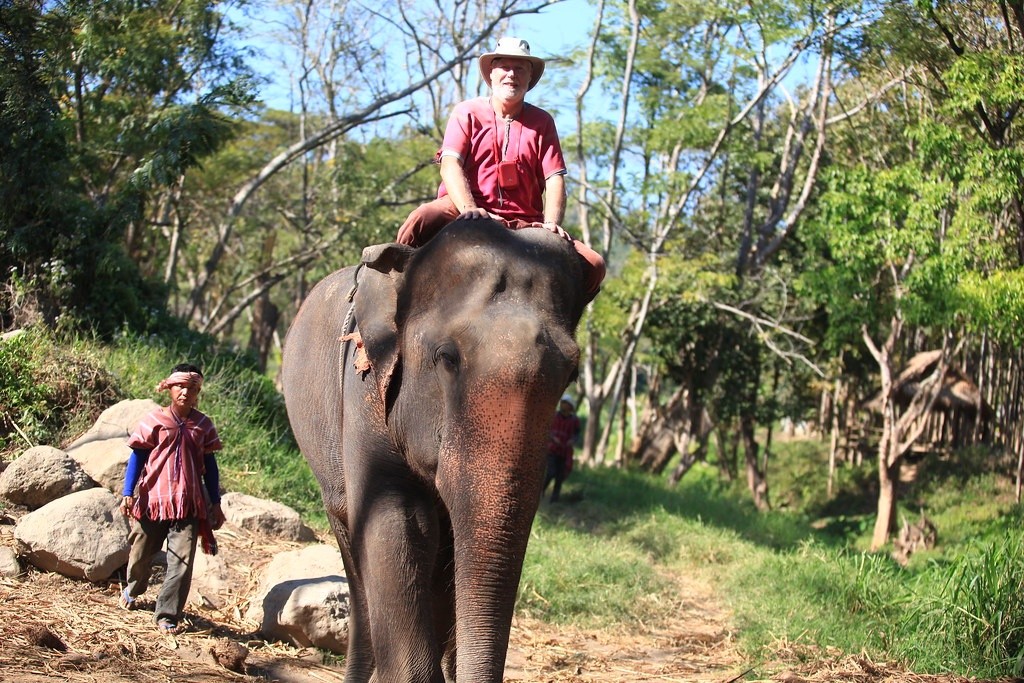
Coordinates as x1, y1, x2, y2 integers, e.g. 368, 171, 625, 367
280, 212, 584, 683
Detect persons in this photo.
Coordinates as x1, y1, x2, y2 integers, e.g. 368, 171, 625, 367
120, 363, 225, 636
398, 36, 606, 300
541, 396, 580, 503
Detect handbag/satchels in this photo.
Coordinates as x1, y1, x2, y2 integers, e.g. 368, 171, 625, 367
200, 475, 218, 531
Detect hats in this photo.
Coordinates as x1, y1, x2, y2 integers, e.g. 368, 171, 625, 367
478, 37, 545, 92
561, 393, 576, 411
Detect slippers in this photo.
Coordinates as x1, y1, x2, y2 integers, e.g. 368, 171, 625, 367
158, 617, 179, 635
119, 585, 137, 610
